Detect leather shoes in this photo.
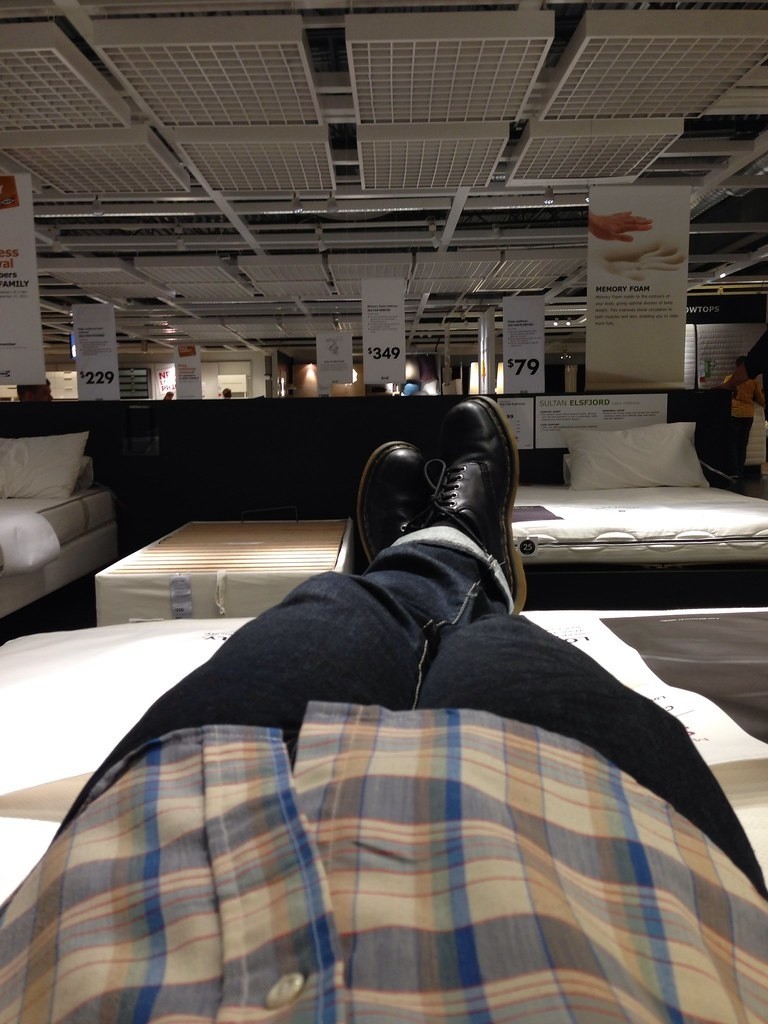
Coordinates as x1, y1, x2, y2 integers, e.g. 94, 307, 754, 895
356, 439, 436, 563
402, 394, 527, 615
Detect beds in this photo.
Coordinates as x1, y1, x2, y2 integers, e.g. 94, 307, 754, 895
511, 485, 768, 611
0, 609, 768, 902
0, 489, 117, 620
94, 519, 351, 625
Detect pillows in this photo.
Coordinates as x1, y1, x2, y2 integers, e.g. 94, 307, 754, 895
560, 422, 708, 492
0, 431, 89, 499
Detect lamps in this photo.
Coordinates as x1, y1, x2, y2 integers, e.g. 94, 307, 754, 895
584, 185, 589, 203
291, 191, 303, 214
90, 194, 103, 215
543, 186, 553, 204
175, 235, 186, 250
314, 225, 328, 252
325, 190, 338, 214
491, 223, 503, 237
428, 223, 440, 248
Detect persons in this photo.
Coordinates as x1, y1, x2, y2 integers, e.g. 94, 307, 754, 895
17, 378, 53, 402
590, 210, 653, 241
599, 243, 685, 280
179, 347, 186, 355
723, 355, 763, 477
223, 388, 232, 399
288, 389, 294, 397
711, 331, 768, 422
403, 372, 438, 395
0, 392, 768, 1024
163, 392, 174, 400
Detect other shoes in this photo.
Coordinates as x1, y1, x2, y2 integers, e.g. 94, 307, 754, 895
730, 474, 746, 478
